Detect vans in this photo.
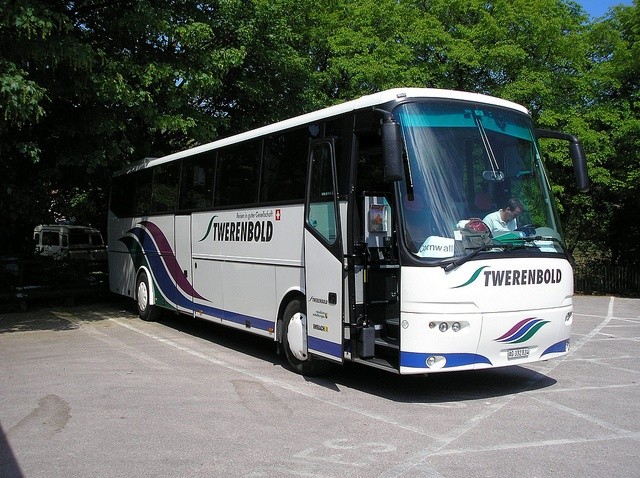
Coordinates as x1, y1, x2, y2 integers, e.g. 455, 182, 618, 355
33, 223, 108, 271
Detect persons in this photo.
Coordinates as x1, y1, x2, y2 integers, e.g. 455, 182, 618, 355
483, 199, 523, 236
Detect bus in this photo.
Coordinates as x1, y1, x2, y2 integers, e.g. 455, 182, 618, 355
106, 87, 590, 379
